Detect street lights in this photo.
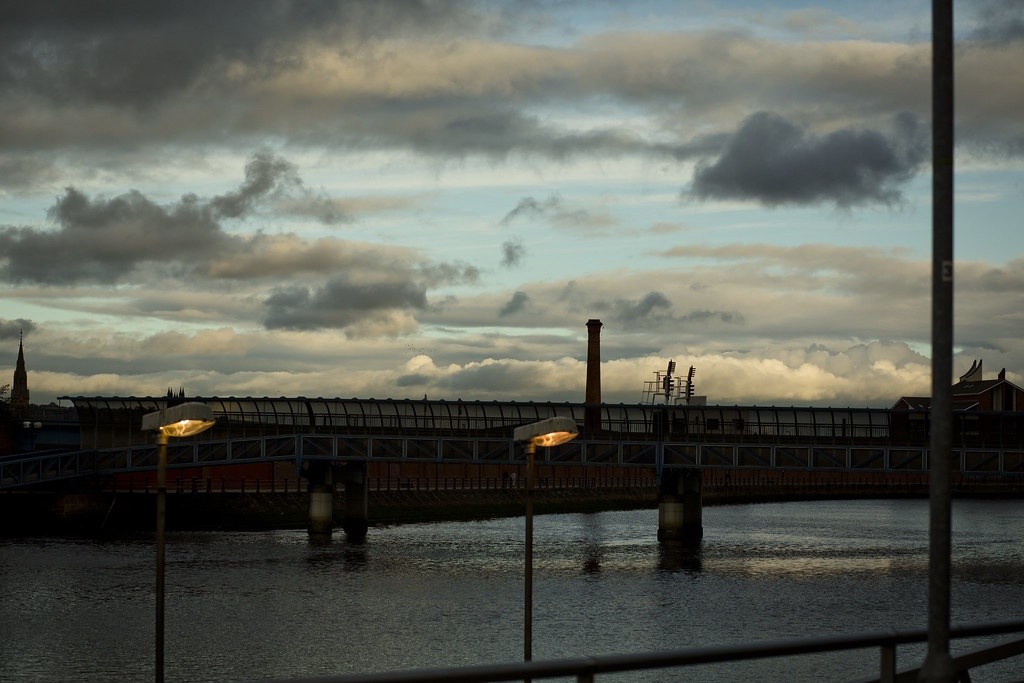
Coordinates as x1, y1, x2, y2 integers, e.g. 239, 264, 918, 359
23, 422, 42, 452
141, 402, 216, 683
512, 417, 586, 683
